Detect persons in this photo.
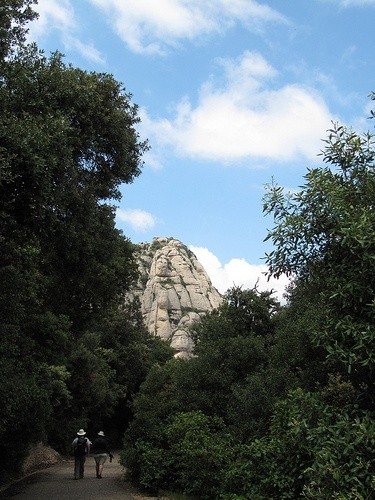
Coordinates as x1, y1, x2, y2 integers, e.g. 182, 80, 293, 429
91, 430, 113, 479
71, 428, 92, 480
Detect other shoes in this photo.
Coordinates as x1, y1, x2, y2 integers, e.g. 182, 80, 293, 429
75, 475, 78, 479
98, 475, 103, 479
96, 473, 98, 477
80, 473, 84, 479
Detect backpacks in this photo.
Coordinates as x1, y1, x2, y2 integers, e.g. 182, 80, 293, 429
77, 438, 87, 455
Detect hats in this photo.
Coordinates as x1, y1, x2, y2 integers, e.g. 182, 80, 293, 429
77, 429, 86, 435
98, 431, 105, 436
